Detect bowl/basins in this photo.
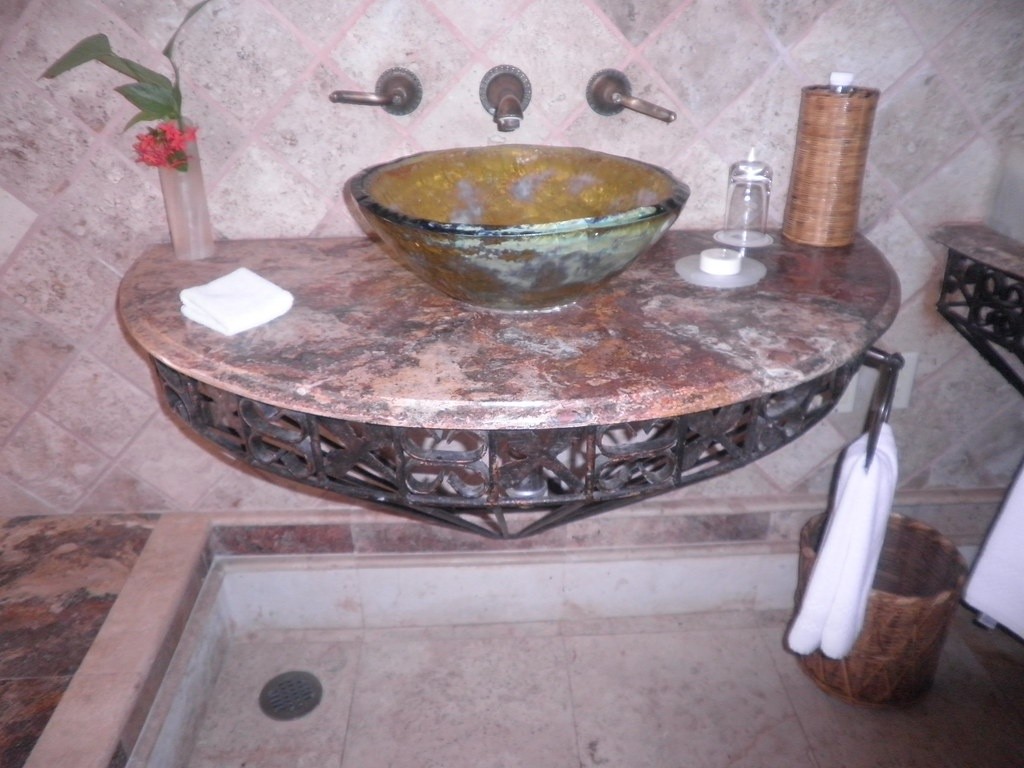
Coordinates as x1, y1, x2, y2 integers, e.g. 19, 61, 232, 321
347, 144, 691, 312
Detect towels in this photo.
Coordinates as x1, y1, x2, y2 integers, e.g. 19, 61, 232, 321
961, 460, 1024, 642
178, 264, 297, 336
785, 421, 901, 662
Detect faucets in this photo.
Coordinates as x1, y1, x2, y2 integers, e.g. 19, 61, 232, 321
477, 63, 532, 133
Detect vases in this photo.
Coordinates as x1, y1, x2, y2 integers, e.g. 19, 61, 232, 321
156, 117, 214, 261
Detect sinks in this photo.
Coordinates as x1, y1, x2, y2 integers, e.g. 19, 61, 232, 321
348, 142, 691, 311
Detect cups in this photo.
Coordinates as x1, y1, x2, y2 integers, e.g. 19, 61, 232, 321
725, 161, 772, 243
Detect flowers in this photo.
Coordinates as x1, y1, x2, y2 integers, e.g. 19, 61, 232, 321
37, 1, 210, 171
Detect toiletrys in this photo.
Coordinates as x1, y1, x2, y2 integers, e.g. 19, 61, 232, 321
781, 68, 882, 250
